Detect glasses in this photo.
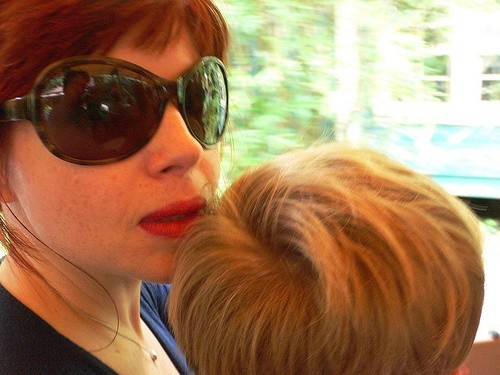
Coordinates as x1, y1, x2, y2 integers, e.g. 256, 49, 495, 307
0, 56, 231, 166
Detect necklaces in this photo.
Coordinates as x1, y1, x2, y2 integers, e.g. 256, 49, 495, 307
47, 278, 159, 363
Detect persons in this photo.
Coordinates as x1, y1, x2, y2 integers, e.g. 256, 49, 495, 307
0, 0, 229, 374
169, 137, 485, 375
47, 70, 104, 159
